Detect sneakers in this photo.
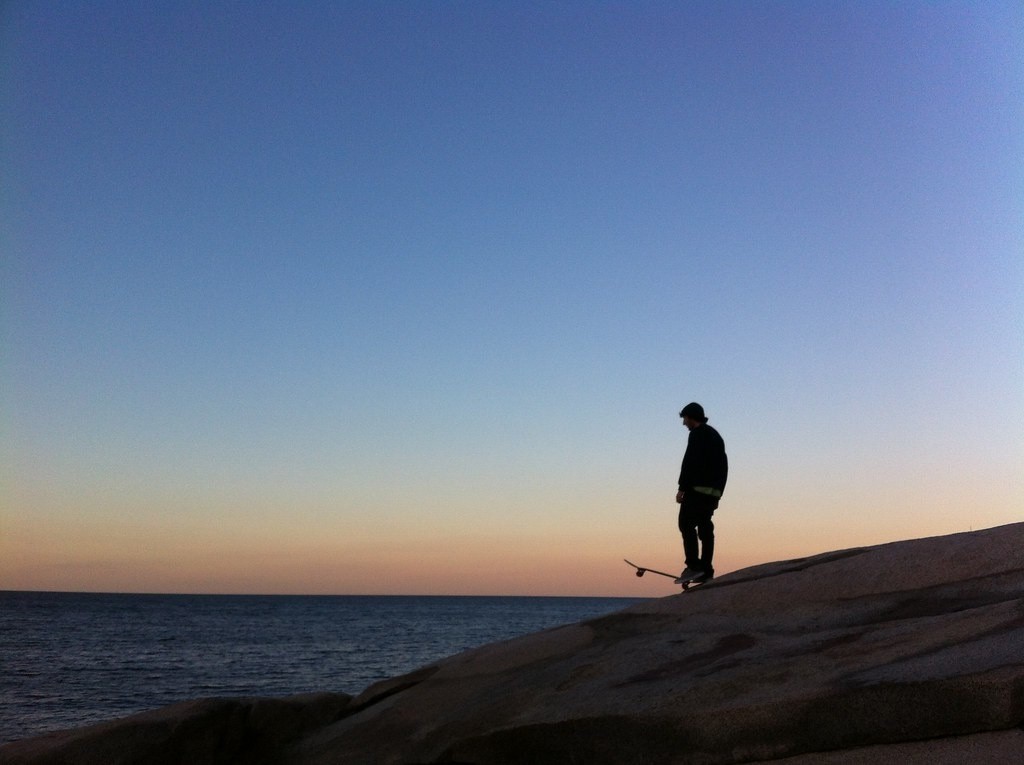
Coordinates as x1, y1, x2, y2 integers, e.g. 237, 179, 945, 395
674, 567, 714, 585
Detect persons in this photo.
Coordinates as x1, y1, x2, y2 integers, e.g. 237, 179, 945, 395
673, 401, 728, 585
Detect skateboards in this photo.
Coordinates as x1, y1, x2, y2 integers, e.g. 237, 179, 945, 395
624, 558, 713, 590
